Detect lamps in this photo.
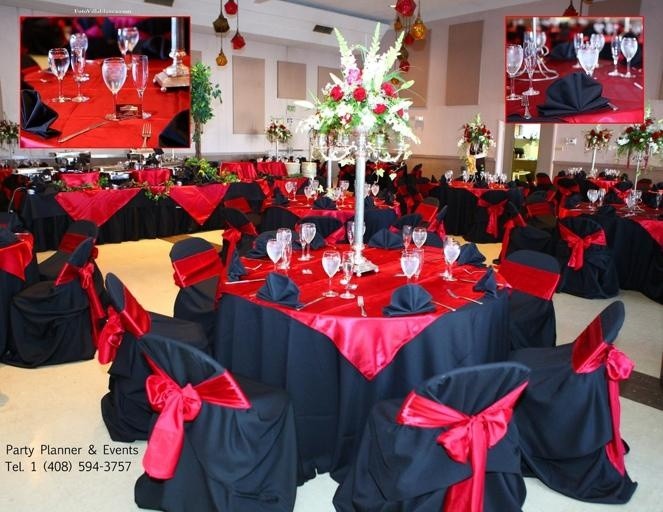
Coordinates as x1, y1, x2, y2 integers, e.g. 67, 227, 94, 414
563, 0, 609, 16
391, 0, 428, 85
213, 0, 246, 66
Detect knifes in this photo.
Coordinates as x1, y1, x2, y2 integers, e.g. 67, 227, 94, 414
224, 278, 263, 285
57, 121, 104, 143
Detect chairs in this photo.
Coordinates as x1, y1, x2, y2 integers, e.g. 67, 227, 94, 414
0, 154, 662, 512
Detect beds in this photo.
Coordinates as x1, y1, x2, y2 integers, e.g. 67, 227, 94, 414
20, 56, 189, 148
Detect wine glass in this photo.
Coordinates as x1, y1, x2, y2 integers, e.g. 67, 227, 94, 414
398, 224, 462, 285
566, 165, 623, 182
508, 28, 638, 99
441, 166, 509, 189
584, 184, 646, 217
284, 178, 381, 208
45, 24, 155, 125
264, 221, 373, 299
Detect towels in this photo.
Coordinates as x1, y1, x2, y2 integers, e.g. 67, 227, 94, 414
535, 69, 609, 116
550, 41, 575, 59
157, 108, 189, 147
20, 89, 61, 139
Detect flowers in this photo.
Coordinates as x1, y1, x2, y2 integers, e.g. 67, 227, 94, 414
615, 101, 663, 154
267, 123, 293, 143
585, 123, 615, 151
292, 17, 419, 148
456, 111, 496, 154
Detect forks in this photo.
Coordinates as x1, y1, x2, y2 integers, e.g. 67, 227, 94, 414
223, 263, 262, 271
519, 91, 533, 121
141, 122, 151, 148
446, 269, 504, 315
357, 296, 368, 317
